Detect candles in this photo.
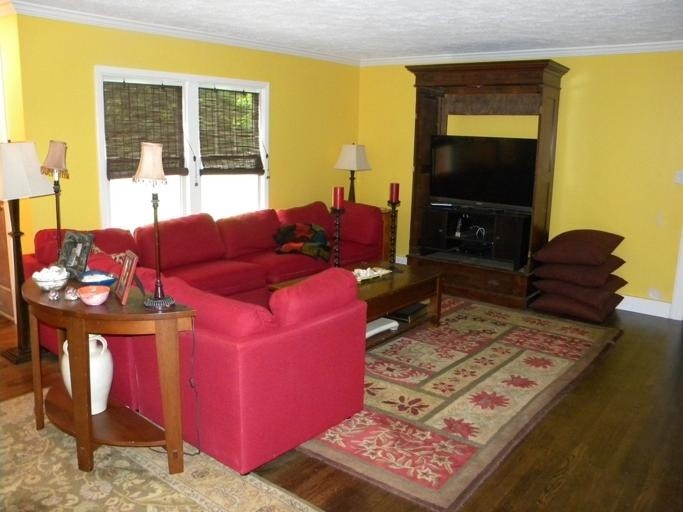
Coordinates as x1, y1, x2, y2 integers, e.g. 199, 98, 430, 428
388, 181, 400, 204
332, 185, 345, 209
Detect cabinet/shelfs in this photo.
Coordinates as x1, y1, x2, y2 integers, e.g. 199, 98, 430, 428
379, 208, 398, 263
405, 58, 573, 311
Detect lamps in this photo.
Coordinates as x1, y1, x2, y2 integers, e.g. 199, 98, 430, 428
130, 140, 177, 311
38, 137, 72, 273
333, 141, 373, 205
1, 139, 58, 366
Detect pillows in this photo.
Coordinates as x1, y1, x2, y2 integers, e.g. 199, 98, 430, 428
525, 225, 631, 323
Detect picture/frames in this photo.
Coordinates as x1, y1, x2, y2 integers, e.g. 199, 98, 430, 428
110, 248, 139, 306
50, 231, 98, 280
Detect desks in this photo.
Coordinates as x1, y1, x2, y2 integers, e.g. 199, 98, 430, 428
19, 260, 196, 476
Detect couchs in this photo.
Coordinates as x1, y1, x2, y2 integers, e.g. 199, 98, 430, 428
14, 197, 383, 477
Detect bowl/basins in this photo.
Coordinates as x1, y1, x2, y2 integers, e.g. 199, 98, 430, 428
76, 284, 109, 306
76, 270, 115, 286
33, 274, 71, 292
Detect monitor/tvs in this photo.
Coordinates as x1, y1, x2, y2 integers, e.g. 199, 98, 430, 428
429, 134, 539, 217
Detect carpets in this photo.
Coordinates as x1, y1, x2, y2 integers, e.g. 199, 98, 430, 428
292, 286, 624, 511
1, 385, 330, 512
